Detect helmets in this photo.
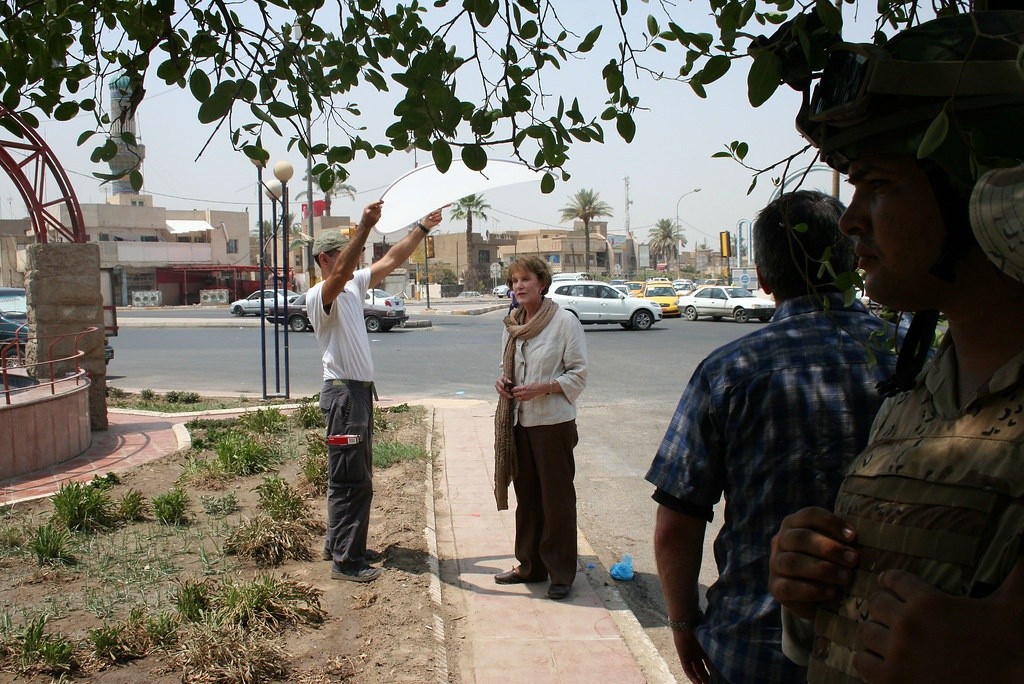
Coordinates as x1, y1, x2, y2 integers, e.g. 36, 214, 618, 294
826, 10, 1024, 195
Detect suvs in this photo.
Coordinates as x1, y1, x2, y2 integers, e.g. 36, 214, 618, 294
544, 280, 663, 330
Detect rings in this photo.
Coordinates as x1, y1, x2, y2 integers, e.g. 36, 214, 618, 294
518, 396, 521, 400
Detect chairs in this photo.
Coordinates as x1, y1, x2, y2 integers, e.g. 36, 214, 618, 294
648, 290, 655, 296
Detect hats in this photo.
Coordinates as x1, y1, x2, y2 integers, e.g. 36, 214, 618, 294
312, 232, 349, 257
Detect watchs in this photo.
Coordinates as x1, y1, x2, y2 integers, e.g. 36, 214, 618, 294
546, 382, 553, 397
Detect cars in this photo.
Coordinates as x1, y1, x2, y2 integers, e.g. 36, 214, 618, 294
672, 278, 723, 296
267, 292, 408, 332
493, 283, 512, 298
365, 290, 406, 313
678, 287, 775, 323
612, 279, 684, 319
457, 291, 479, 296
0, 308, 114, 368
230, 288, 299, 317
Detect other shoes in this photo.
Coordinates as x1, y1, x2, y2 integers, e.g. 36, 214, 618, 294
546, 584, 571, 598
331, 565, 384, 581
495, 569, 549, 584
324, 549, 380, 563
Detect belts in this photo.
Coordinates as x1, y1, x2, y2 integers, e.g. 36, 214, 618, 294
332, 379, 379, 401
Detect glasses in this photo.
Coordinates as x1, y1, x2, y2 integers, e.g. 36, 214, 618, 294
805, 42, 892, 123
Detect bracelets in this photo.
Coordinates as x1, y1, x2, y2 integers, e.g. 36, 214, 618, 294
417, 223, 430, 233
665, 607, 706, 632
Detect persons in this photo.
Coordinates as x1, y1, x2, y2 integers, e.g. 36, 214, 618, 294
767, 12, 1024, 683
494, 254, 588, 598
644, 190, 937, 684
306, 201, 452, 581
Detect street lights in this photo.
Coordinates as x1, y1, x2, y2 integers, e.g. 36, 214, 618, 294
266, 180, 283, 393
251, 148, 271, 399
677, 188, 701, 279
274, 161, 295, 399
